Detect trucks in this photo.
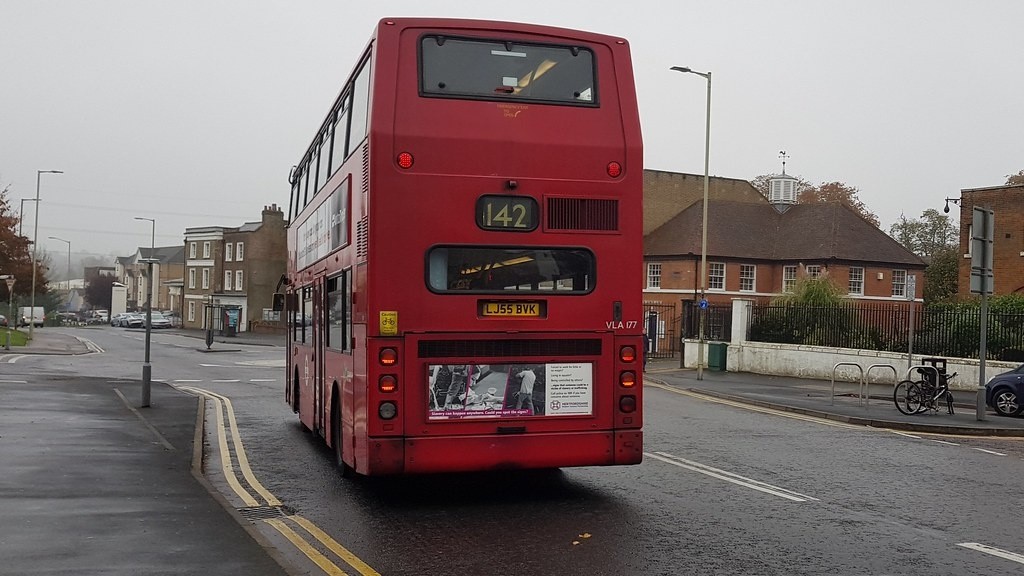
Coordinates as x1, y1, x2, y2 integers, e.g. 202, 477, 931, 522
17, 307, 45, 328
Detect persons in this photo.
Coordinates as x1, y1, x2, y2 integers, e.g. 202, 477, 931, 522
643, 328, 649, 372
514, 366, 536, 416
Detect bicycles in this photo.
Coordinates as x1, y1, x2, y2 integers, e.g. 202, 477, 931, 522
890, 369, 957, 416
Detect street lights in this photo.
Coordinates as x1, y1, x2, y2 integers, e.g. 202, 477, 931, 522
17, 169, 64, 340
133, 214, 153, 406
47, 234, 71, 324
671, 64, 713, 381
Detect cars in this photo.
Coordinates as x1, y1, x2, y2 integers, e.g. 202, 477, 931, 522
985, 364, 1024, 415
85, 308, 182, 330
0, 315, 8, 326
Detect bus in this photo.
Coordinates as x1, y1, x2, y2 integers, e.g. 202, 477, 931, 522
55, 312, 76, 323
268, 16, 646, 489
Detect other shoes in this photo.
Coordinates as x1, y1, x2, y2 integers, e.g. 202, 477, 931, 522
643, 370, 646, 373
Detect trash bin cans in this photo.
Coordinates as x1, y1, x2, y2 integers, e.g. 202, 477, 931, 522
707, 342, 729, 371
921, 356, 947, 397
225, 323, 237, 337
